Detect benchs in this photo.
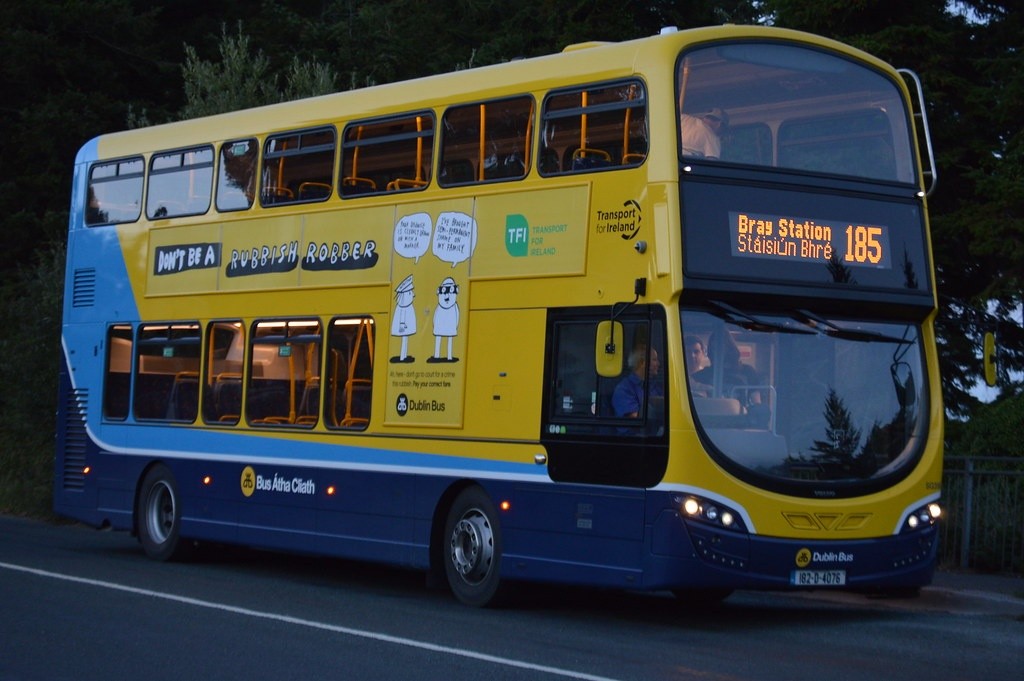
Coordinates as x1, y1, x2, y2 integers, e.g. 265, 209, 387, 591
105, 319, 374, 430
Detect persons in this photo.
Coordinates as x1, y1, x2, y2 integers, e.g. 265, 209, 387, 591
681, 108, 731, 158
615, 330, 758, 419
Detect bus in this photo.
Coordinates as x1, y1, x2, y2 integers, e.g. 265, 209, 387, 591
55, 26, 1000, 614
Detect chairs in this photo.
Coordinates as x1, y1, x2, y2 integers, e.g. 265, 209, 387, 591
87, 148, 648, 221
